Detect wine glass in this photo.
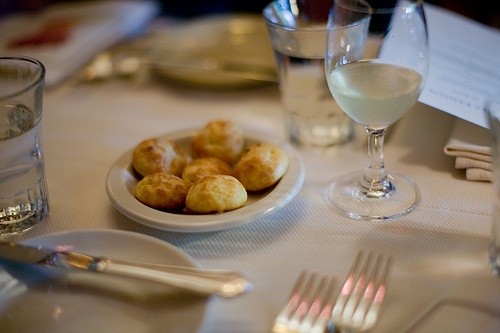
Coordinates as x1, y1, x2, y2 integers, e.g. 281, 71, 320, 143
324, 2, 429, 221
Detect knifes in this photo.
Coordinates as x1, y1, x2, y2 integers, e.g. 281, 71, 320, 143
0, 241, 254, 299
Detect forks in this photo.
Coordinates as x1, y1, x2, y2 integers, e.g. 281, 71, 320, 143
271, 270, 335, 333
332, 250, 392, 333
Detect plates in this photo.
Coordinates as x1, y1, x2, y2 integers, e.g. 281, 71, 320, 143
106, 124, 305, 234
149, 13, 280, 91
0, 229, 209, 333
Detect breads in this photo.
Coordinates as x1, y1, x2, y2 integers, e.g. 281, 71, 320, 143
132, 118, 288, 213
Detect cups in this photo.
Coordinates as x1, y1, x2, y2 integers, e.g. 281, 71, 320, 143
262, 1, 372, 147
484, 96, 500, 275
0, 53, 51, 235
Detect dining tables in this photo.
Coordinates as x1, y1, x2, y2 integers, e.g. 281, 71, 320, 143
0, 0, 500, 333
